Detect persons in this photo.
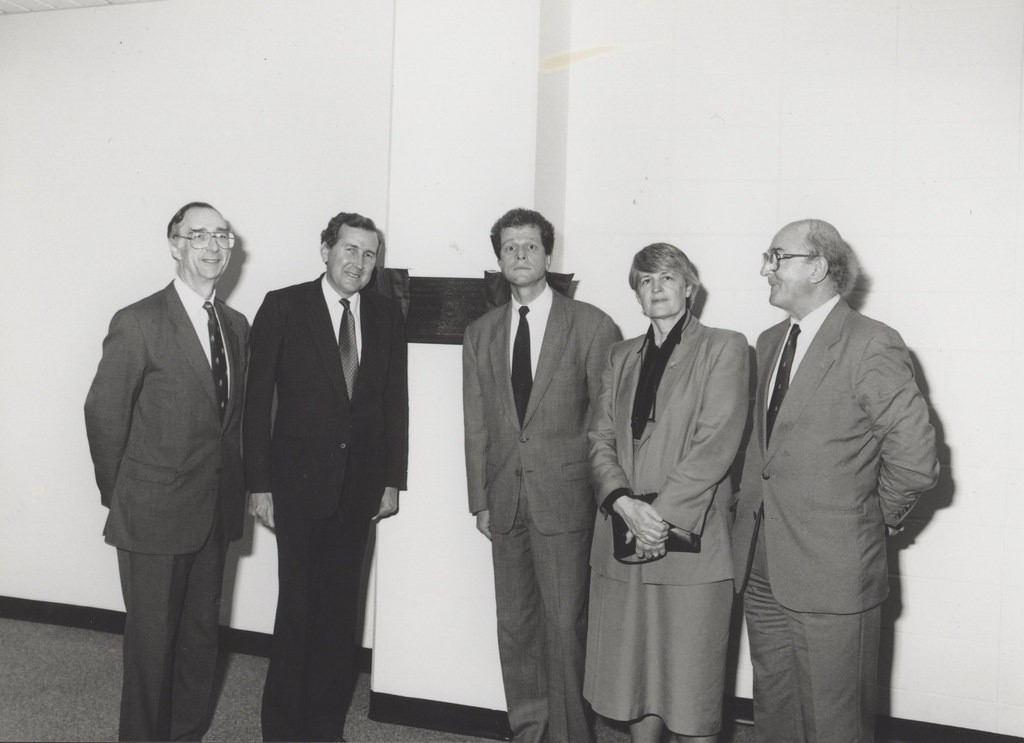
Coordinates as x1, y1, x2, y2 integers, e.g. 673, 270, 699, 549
249, 212, 409, 743
462, 208, 625, 743
582, 243, 749, 743
731, 219, 939, 743
84, 202, 251, 743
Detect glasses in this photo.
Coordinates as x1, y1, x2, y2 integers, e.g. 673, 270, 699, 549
173, 231, 235, 250
762, 251, 816, 272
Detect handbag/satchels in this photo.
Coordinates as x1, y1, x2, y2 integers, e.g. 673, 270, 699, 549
611, 493, 702, 564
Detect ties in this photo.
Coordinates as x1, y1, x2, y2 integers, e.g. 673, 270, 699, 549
339, 298, 359, 401
203, 301, 228, 427
765, 323, 801, 445
511, 305, 534, 429
